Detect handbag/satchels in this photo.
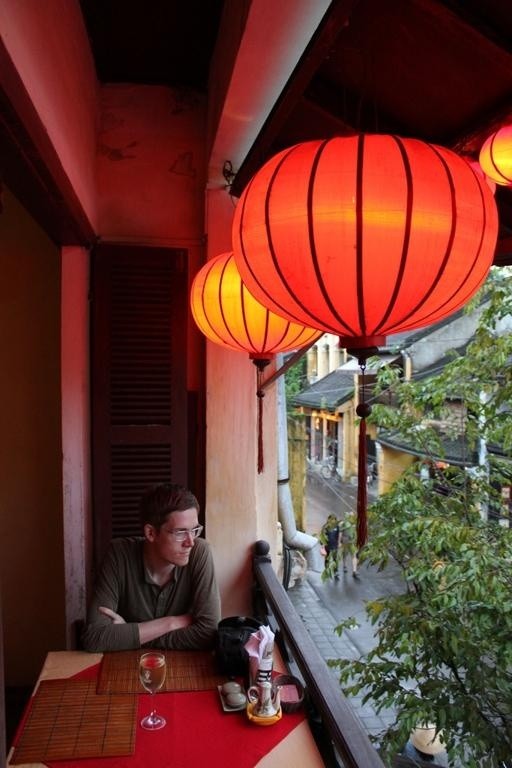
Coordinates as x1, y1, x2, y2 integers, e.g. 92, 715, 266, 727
319, 548, 327, 557
212, 615, 265, 679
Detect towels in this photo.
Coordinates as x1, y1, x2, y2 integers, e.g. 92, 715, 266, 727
242, 623, 276, 663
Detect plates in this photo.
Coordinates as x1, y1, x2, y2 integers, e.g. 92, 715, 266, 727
217, 685, 247, 712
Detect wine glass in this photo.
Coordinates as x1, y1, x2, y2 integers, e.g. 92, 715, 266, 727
139, 652, 167, 731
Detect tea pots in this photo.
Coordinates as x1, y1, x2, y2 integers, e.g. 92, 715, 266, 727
248, 682, 283, 717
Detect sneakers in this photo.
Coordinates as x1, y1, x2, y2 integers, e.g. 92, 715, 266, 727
335, 568, 360, 581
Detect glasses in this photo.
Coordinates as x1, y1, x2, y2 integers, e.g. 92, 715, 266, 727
159, 524, 204, 542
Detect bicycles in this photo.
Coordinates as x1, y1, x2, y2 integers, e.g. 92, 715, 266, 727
322, 458, 341, 482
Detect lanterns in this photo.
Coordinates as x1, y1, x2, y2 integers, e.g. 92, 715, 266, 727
477, 118, 512, 189
185, 248, 325, 476
227, 123, 508, 552
442, 134, 500, 198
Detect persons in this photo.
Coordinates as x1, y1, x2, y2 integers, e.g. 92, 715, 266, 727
366, 461, 380, 480
75, 481, 226, 657
366, 471, 373, 487
337, 511, 361, 580
319, 511, 341, 580
